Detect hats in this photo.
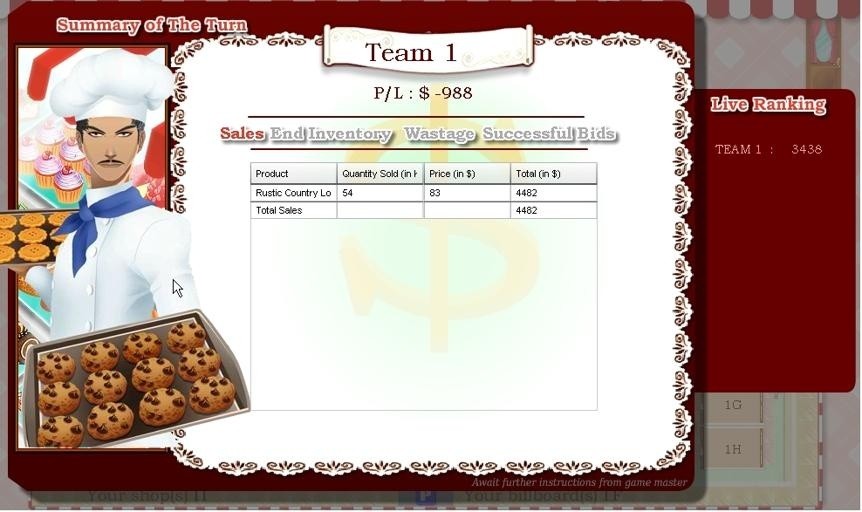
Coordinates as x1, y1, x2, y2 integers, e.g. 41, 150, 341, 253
46, 49, 174, 123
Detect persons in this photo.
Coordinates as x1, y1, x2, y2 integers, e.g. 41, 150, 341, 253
23, 48, 199, 341
